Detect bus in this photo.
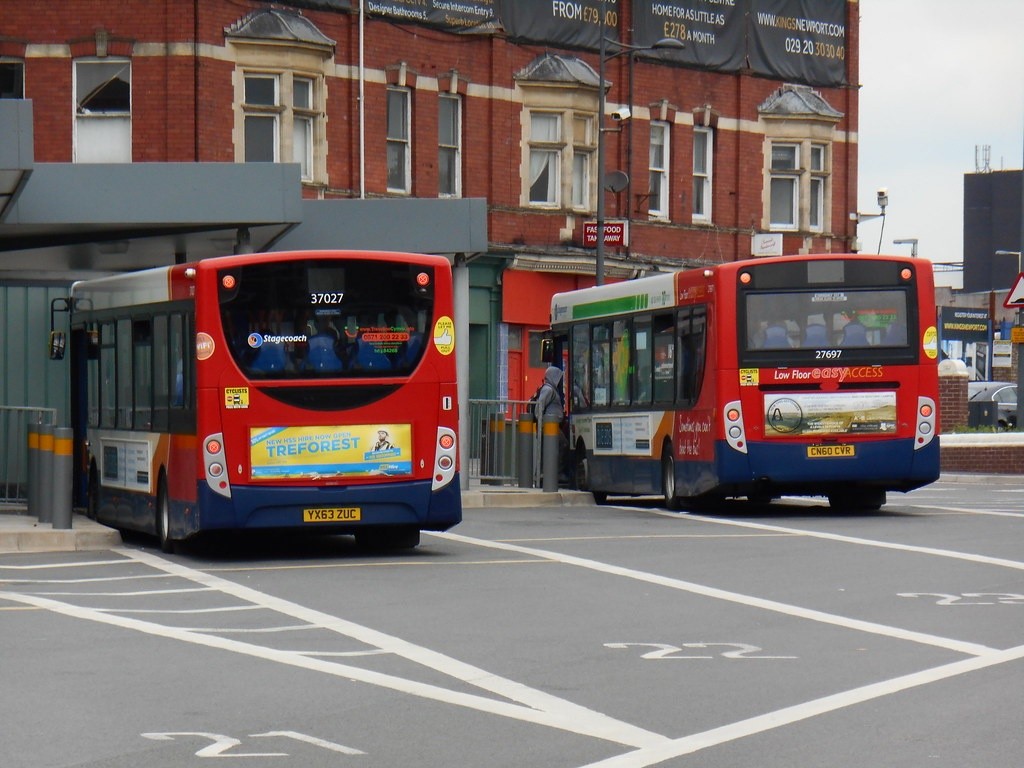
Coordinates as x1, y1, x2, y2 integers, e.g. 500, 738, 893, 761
539, 253, 940, 513
47, 248, 463, 556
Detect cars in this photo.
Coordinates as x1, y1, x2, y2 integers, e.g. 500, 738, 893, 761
967, 380, 1019, 432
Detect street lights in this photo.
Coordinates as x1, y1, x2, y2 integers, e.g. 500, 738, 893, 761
995, 250, 1022, 275
893, 238, 918, 258
594, 0, 687, 288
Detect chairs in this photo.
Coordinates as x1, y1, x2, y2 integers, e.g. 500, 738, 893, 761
299, 335, 341, 371
348, 337, 393, 376
396, 332, 423, 368
882, 321, 906, 345
799, 325, 830, 346
840, 322, 869, 346
252, 338, 285, 371
762, 325, 791, 349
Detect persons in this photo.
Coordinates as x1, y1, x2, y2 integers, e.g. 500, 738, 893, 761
373, 428, 395, 450
534, 366, 570, 486
